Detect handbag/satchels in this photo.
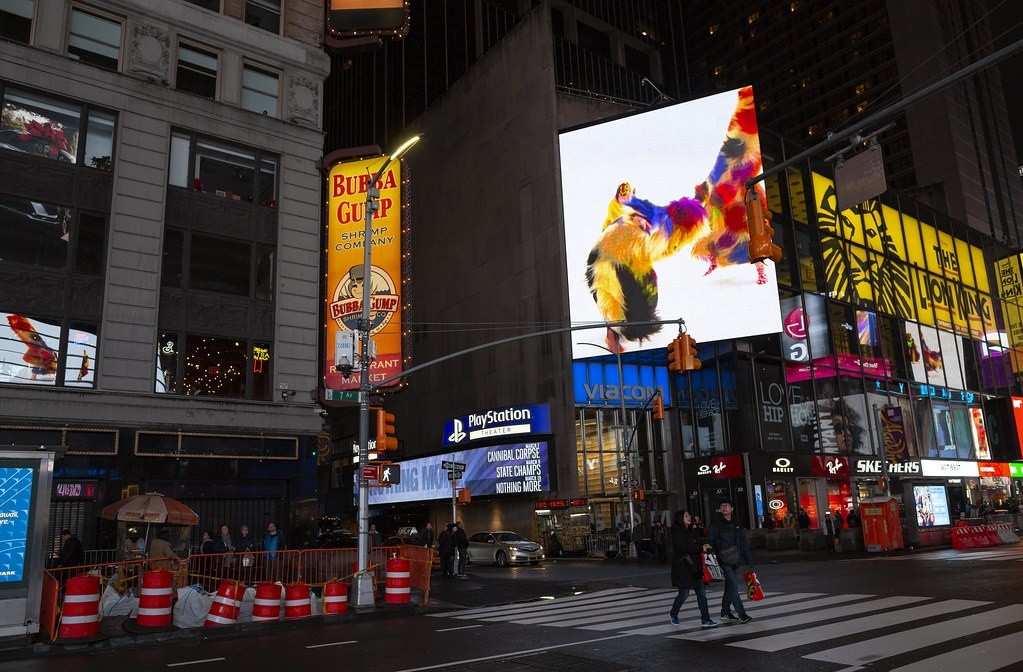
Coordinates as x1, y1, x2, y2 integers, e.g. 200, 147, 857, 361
702, 548, 722, 583
719, 545, 738, 562
743, 570, 764, 600
242, 547, 255, 567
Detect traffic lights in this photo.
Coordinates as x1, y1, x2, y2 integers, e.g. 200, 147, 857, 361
669, 337, 683, 374
457, 490, 472, 503
377, 462, 402, 485
680, 332, 700, 372
373, 407, 399, 450
653, 396, 664, 419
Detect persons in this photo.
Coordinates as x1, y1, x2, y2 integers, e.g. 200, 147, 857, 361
122, 527, 146, 585
438, 523, 456, 577
667, 509, 720, 629
453, 520, 471, 580
235, 524, 255, 580
58, 528, 83, 569
822, 513, 836, 553
633, 514, 708, 565
148, 529, 178, 569
784, 510, 796, 528
215, 523, 236, 578
801, 378, 866, 456
764, 513, 775, 529
367, 524, 382, 552
845, 493, 1023, 528
798, 508, 811, 529
200, 530, 216, 571
585, 182, 710, 354
832, 509, 844, 543
707, 498, 755, 623
262, 522, 285, 580
419, 522, 435, 576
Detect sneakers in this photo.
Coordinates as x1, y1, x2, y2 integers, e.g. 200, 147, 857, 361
703, 619, 717, 627
720, 612, 739, 620
668, 611, 680, 626
740, 615, 750, 624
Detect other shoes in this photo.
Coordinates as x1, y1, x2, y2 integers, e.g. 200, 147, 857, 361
445, 572, 455, 575
457, 574, 470, 580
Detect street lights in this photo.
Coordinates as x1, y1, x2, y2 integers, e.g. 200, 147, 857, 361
348, 131, 424, 613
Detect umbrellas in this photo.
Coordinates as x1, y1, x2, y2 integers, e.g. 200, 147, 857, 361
99, 491, 200, 553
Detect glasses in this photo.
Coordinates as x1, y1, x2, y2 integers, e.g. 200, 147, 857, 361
683, 515, 691, 518
720, 505, 731, 509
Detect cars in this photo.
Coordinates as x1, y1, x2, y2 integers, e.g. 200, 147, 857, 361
456, 531, 546, 566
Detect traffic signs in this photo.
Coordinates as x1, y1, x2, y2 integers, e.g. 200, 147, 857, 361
448, 472, 463, 481
441, 460, 465, 472
